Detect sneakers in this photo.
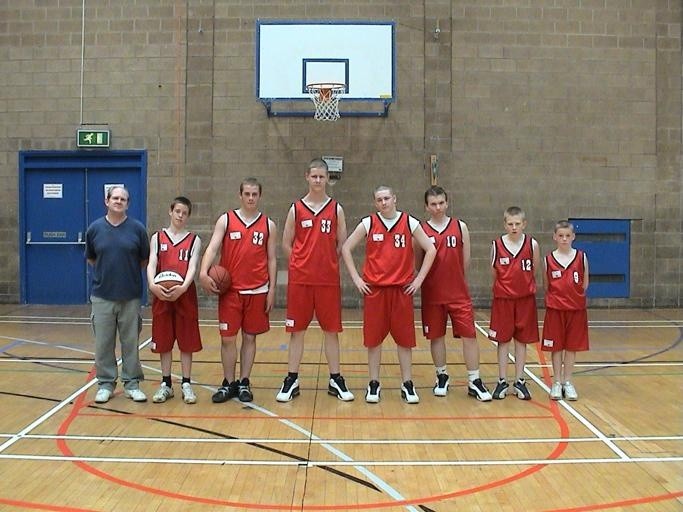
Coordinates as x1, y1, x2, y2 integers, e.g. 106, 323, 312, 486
212, 378, 253, 402
152, 382, 174, 403
433, 374, 449, 396
276, 377, 300, 402
549, 381, 577, 401
493, 378, 509, 400
328, 376, 354, 401
181, 382, 196, 404
365, 380, 381, 403
513, 377, 531, 400
95, 389, 113, 403
401, 380, 419, 404
123, 389, 147, 402
467, 378, 492, 402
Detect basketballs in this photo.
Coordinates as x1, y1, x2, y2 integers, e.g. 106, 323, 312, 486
207, 265, 232, 296
153, 271, 184, 294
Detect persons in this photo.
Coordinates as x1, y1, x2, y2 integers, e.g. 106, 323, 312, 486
540, 220, 589, 400
489, 207, 539, 400
83, 186, 151, 404
275, 158, 354, 403
199, 178, 277, 403
341, 185, 437, 404
412, 185, 493, 402
146, 196, 202, 405
